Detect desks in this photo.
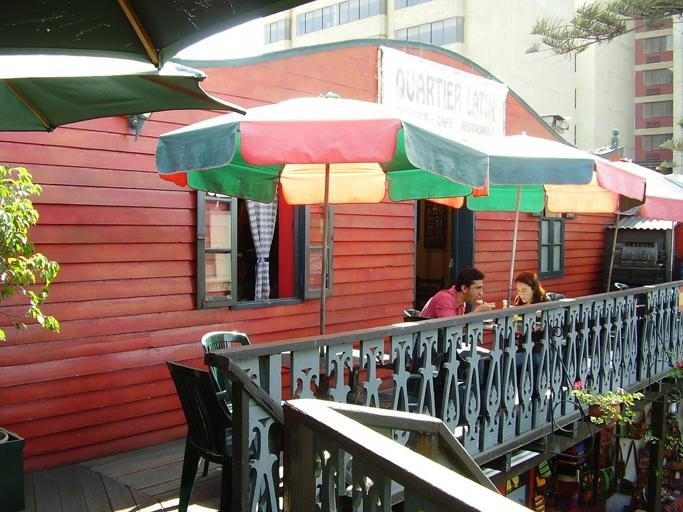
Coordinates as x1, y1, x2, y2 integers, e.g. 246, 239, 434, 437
279, 346, 396, 406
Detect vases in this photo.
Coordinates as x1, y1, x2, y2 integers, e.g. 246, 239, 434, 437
587, 403, 620, 428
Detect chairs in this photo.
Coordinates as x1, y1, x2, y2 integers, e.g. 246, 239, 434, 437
162, 331, 278, 512
393, 308, 452, 418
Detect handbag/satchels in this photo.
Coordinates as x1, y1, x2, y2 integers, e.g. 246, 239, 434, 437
616, 448, 625, 478
620, 479, 644, 497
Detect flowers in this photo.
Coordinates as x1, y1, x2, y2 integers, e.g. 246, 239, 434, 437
569, 379, 644, 425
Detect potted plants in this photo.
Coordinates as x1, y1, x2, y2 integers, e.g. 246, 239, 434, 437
0, 161, 61, 509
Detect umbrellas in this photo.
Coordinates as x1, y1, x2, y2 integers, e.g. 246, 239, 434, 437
606, 157, 683, 292
419, 124, 648, 310
0, 49, 246, 133
0, 0, 317, 68
154, 92, 490, 336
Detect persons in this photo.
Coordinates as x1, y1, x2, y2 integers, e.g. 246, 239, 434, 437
509, 271, 550, 303
420, 266, 496, 318
